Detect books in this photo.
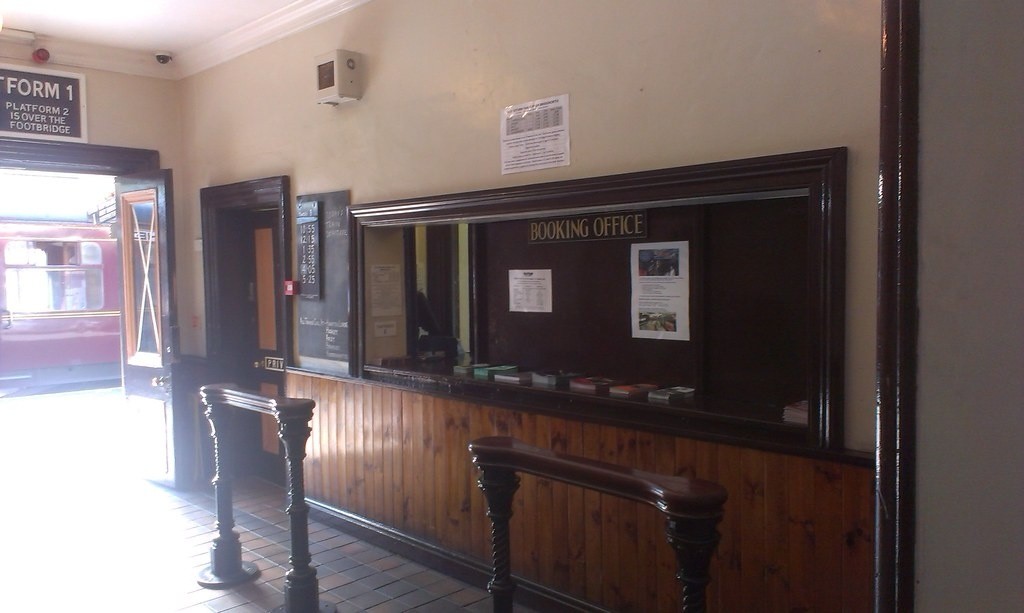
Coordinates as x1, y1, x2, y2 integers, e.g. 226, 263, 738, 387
454, 359, 695, 400
783, 400, 808, 424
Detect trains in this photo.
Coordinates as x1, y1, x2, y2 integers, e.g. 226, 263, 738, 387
0, 208, 119, 390
